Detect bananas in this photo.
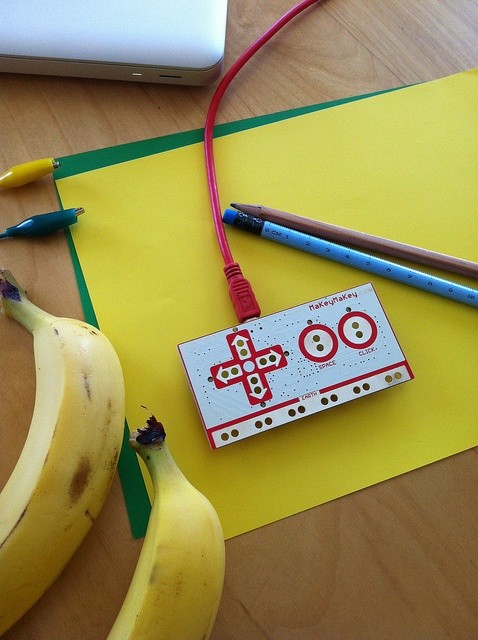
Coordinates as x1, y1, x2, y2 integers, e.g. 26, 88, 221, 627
104, 417, 228, 639
1, 268, 128, 640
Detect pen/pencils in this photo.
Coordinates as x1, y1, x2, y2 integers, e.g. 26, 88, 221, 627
223, 210, 478, 324
228, 201, 477, 283
